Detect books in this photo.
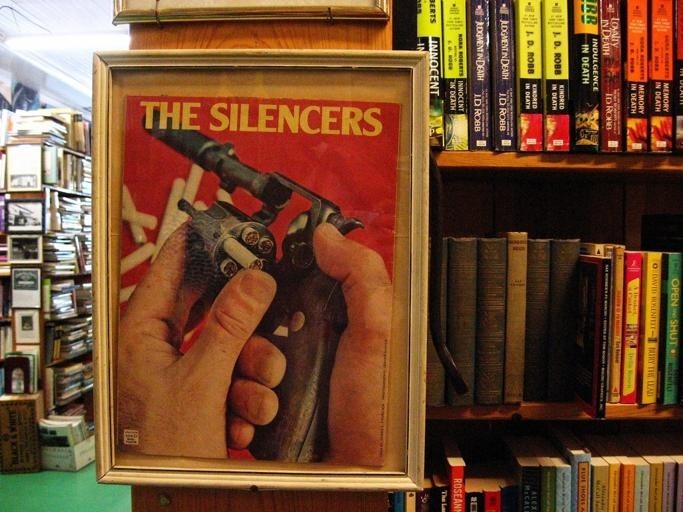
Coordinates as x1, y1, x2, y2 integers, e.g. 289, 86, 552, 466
0, 105, 95, 474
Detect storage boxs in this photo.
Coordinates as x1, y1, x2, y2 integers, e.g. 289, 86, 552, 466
0, 389, 96, 475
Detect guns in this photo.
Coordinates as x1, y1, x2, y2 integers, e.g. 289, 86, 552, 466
141, 110, 366, 464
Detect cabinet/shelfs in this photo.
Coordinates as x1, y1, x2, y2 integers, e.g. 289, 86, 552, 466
128, 1, 683, 512
0, 134, 95, 434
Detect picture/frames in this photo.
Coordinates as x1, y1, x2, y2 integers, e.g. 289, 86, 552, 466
91, 49, 431, 497
5, 142, 45, 379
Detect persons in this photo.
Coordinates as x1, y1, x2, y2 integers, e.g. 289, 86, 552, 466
117, 204, 391, 470
